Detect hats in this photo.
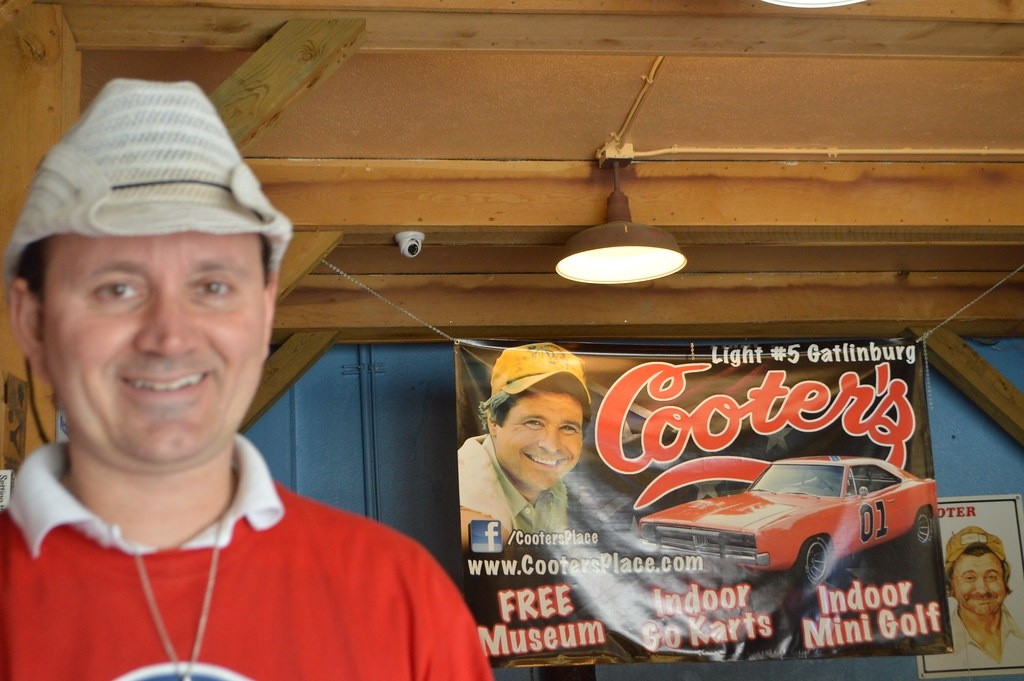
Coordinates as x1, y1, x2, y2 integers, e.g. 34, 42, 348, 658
2, 76, 291, 288
944, 526, 1006, 565
490, 341, 595, 411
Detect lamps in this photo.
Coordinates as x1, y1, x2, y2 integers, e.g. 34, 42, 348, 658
555, 161, 688, 285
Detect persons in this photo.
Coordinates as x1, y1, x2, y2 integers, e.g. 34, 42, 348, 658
0, 78, 493, 681
457, 344, 593, 549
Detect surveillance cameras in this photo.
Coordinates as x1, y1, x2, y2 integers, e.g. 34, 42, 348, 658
396, 232, 424, 258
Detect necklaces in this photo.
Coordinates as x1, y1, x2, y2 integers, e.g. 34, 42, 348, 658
135, 520, 222, 681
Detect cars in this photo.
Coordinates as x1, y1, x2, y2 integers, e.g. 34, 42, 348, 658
637, 455, 937, 590
